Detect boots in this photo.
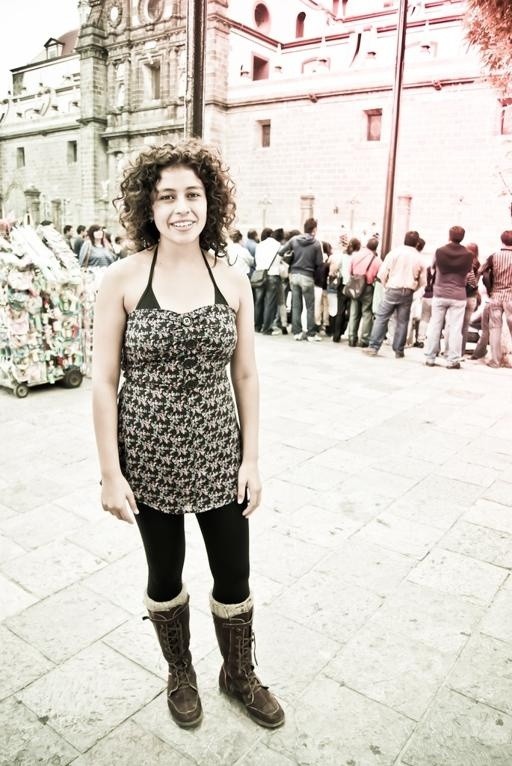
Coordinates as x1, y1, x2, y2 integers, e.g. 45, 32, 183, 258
207, 586, 287, 728
142, 582, 203, 729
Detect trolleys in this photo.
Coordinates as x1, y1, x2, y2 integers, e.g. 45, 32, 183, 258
1, 349, 83, 399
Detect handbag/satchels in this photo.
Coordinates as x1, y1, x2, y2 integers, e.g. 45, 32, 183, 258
341, 273, 367, 300
249, 269, 270, 289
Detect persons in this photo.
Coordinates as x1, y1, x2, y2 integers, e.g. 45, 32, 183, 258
91, 141, 286, 730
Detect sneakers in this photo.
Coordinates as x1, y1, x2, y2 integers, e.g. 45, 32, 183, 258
255, 325, 461, 369
